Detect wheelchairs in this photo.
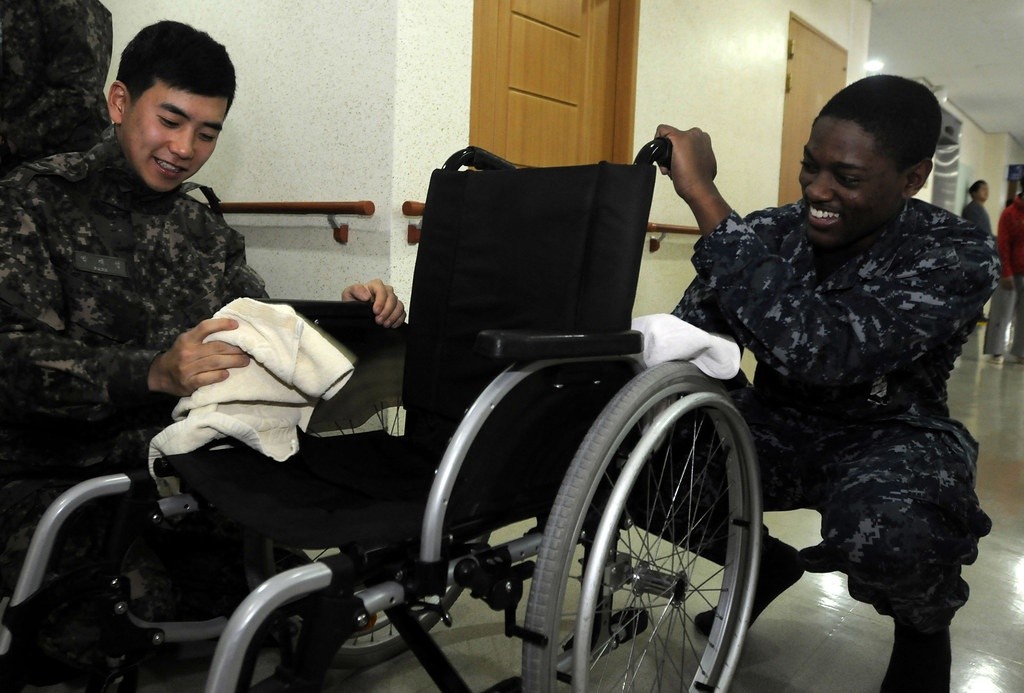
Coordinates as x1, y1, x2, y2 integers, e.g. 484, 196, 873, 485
0, 134, 763, 693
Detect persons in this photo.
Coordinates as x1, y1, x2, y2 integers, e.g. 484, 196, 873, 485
655, 73, 1002, 693
0, 19, 407, 675
961, 165, 1024, 365
0, 1, 114, 175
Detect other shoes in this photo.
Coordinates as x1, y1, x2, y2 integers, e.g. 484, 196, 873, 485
880, 621, 950, 693
695, 536, 804, 636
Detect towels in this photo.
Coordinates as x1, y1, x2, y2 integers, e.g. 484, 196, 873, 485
147, 297, 356, 498
630, 314, 741, 453
